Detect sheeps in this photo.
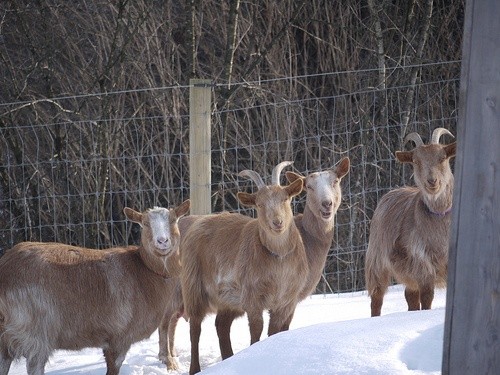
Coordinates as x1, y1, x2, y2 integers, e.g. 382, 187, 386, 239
362, 128, 456, 315
184, 161, 307, 369
0, 198, 194, 374
179, 157, 350, 305
